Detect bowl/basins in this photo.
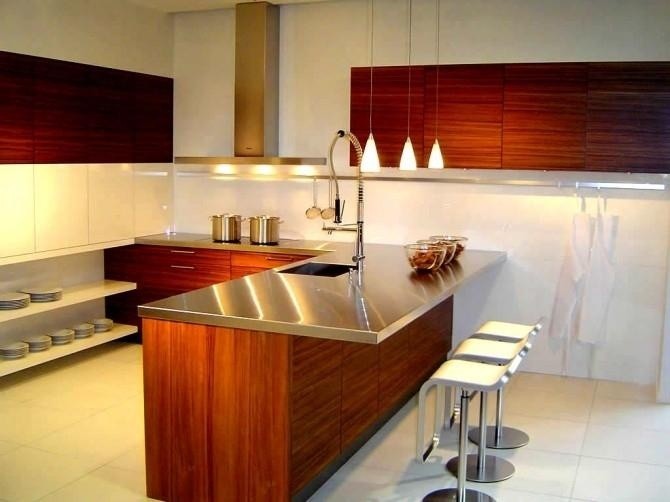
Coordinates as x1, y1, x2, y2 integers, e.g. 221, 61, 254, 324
403, 235, 469, 272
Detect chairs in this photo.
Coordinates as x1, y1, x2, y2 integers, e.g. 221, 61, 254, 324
415, 314, 547, 501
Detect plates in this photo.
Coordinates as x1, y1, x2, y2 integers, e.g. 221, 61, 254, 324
0, 318, 114, 360
1, 286, 62, 311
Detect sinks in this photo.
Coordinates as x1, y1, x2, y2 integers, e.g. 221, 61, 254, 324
276, 259, 358, 279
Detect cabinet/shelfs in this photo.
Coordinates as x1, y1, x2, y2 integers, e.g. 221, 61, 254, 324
424, 63, 502, 170
584, 60, 670, 174
0, 49, 174, 163
102, 245, 316, 343
502, 61, 584, 172
350, 65, 424, 169
0, 164, 177, 254
0, 277, 140, 377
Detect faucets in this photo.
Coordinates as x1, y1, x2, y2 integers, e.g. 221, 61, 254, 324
322, 128, 366, 277
349, 282, 375, 331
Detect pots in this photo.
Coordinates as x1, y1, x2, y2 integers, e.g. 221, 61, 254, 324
207, 213, 286, 246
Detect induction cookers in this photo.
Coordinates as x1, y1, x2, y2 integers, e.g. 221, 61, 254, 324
204, 235, 292, 249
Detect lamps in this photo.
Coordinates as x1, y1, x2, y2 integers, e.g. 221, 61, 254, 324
359, 0, 444, 173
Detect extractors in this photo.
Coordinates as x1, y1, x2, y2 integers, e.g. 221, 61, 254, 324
172, 2, 328, 166
